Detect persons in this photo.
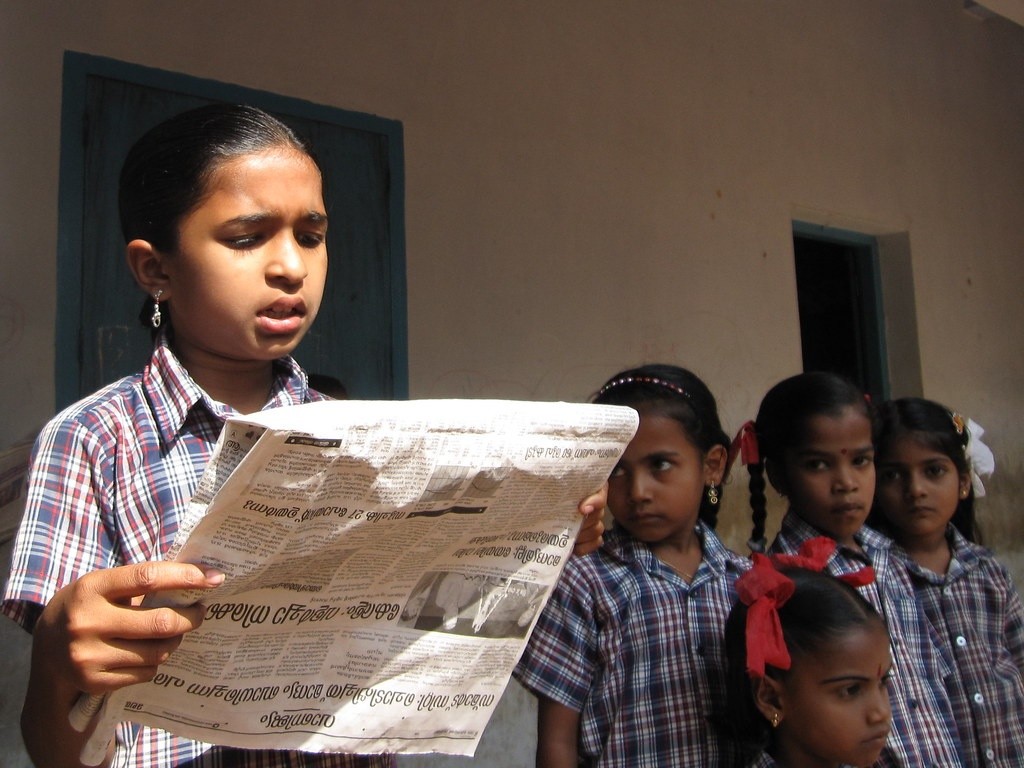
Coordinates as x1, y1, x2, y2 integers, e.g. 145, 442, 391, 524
742, 372, 968, 768
400, 572, 548, 630
512, 363, 754, 768
872, 395, 1024, 768
0, 104, 610, 768
722, 563, 894, 768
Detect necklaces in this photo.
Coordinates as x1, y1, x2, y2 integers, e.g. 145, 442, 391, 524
658, 558, 693, 579
